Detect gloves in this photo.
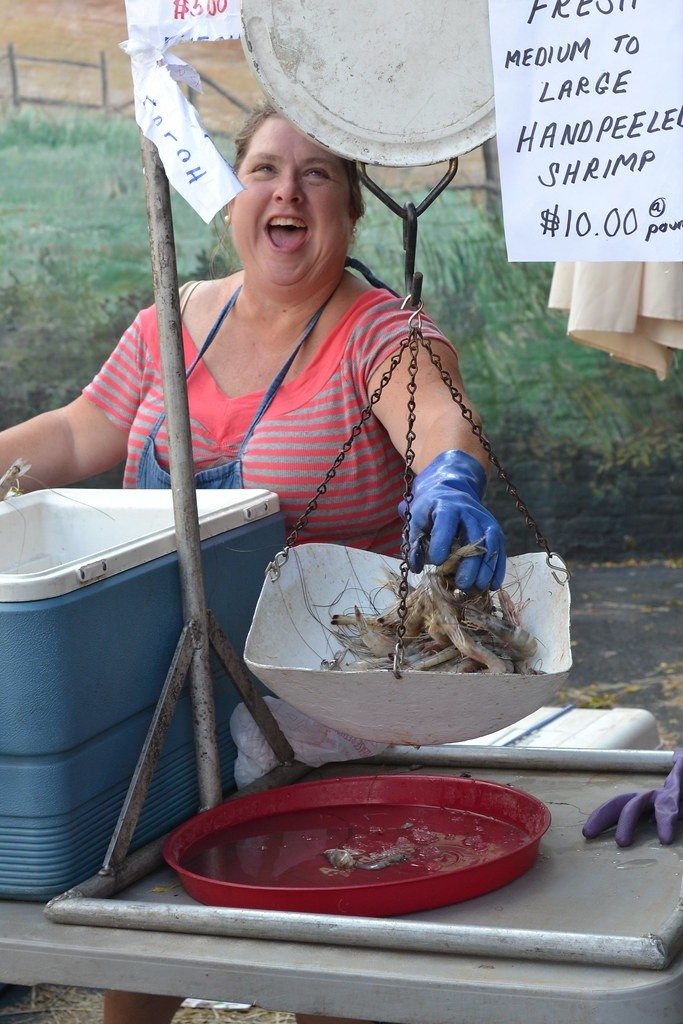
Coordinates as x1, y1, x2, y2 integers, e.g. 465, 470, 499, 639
582, 748, 683, 847
397, 450, 507, 592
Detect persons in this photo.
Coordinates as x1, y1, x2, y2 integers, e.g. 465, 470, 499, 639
2, 98, 507, 593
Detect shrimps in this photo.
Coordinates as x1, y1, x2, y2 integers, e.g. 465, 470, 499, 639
321, 533, 547, 675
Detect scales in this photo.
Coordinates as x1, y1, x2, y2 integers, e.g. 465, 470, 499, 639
233, 0, 577, 744
